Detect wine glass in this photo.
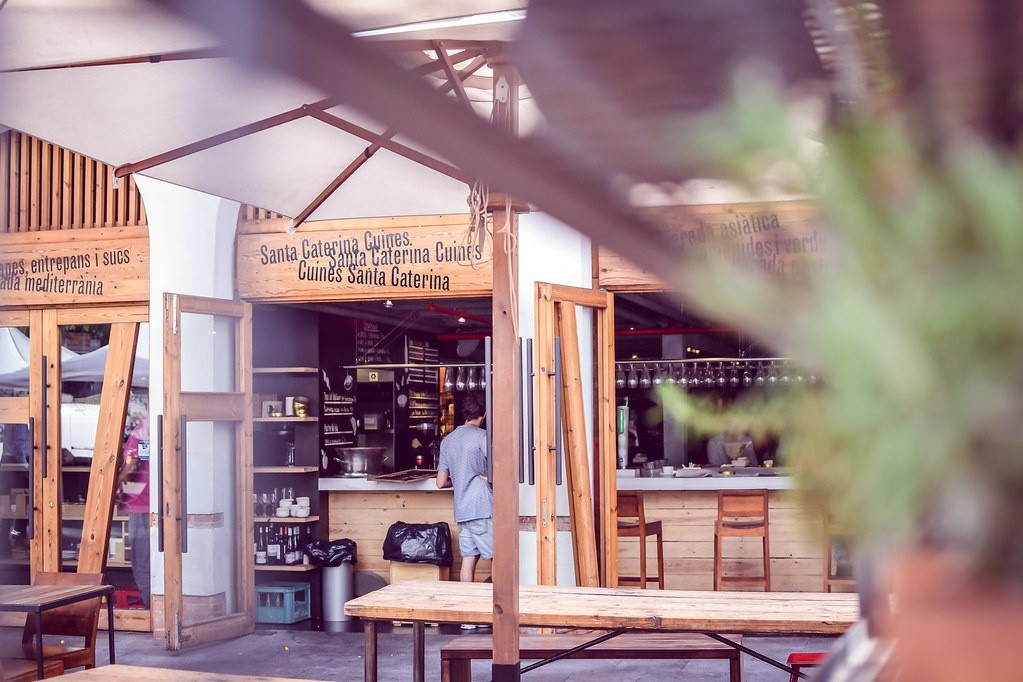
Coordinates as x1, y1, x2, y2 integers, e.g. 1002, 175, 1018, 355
443, 366, 821, 390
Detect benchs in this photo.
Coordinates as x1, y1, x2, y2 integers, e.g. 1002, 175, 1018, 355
786, 652, 832, 682
439, 634, 746, 682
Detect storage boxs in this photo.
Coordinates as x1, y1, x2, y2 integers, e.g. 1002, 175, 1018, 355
255, 580, 311, 624
352, 369, 394, 383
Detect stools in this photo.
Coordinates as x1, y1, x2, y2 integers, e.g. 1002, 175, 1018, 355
820, 504, 857, 593
713, 490, 772, 592
617, 490, 665, 590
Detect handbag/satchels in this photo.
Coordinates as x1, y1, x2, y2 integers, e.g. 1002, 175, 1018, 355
383, 521, 454, 567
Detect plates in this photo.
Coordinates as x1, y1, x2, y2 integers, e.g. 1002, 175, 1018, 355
661, 472, 676, 476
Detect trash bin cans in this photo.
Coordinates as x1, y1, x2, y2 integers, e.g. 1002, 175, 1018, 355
323, 538, 353, 622
391, 523, 450, 628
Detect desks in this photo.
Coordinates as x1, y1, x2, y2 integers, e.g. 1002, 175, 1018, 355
344, 579, 860, 682
0, 584, 115, 680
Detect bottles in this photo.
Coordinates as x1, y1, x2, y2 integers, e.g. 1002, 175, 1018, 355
254, 525, 312, 566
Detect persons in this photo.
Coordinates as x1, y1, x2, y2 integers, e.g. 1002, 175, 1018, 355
0, 391, 28, 538
436, 393, 494, 630
628, 405, 758, 466
113, 403, 150, 611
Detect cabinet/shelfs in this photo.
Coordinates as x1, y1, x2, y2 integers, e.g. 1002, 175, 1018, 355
253, 366, 320, 571
0, 464, 131, 568
323, 384, 438, 474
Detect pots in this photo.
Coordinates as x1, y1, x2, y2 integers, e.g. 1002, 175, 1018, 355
333, 446, 388, 476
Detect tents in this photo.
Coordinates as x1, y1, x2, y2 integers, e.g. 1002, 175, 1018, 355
0, 322, 150, 393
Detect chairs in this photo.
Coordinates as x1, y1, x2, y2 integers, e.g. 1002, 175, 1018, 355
0, 571, 104, 682
0, 658, 65, 682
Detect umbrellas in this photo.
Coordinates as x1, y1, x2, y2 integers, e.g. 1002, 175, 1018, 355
0, 0, 792, 682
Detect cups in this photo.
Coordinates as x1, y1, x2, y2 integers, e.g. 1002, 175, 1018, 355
663, 466, 674, 473
731, 460, 746, 466
275, 496, 310, 518
